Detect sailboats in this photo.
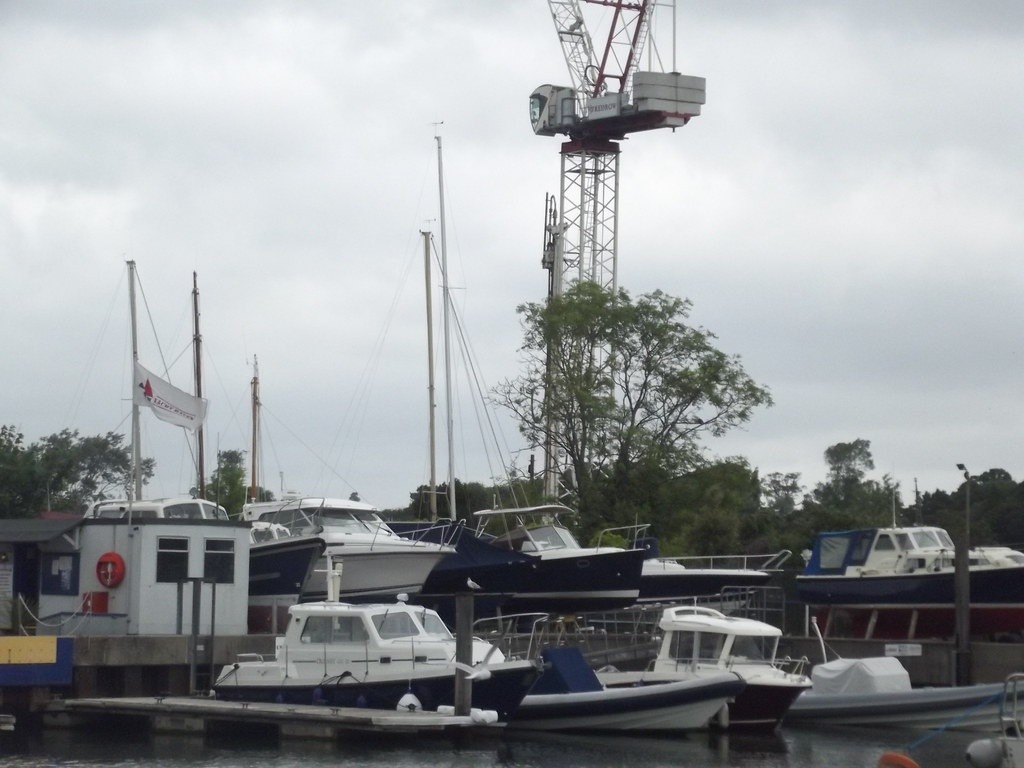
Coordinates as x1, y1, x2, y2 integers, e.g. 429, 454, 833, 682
78, 130, 790, 596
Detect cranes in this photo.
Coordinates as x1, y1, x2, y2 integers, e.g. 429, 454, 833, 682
530, 0, 708, 542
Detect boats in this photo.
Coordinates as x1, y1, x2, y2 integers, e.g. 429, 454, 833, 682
794, 479, 1024, 603
35, 595, 547, 736
777, 617, 1023, 741
482, 595, 818, 736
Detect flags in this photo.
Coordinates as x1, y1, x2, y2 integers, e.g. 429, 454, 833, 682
133, 361, 207, 433
